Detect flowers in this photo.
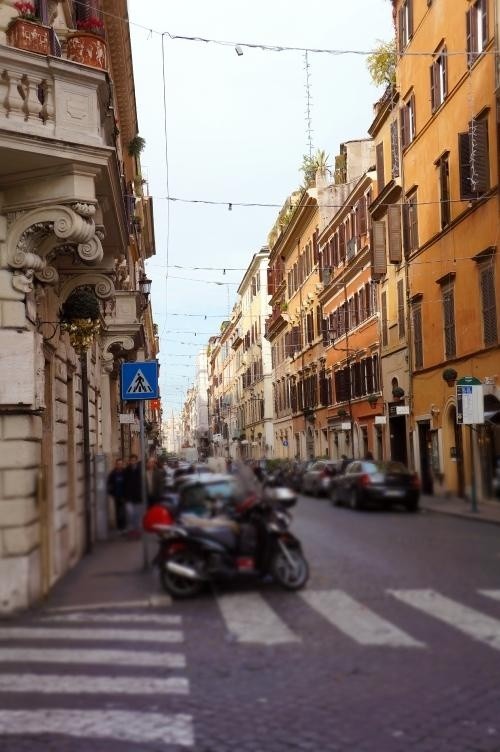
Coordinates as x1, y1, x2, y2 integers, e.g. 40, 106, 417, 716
7, 0, 42, 27
66, 15, 105, 36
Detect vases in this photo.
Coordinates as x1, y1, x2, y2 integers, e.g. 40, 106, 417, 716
4, 17, 52, 55
66, 32, 108, 70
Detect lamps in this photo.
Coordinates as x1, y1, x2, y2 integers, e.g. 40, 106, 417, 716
328, 324, 357, 352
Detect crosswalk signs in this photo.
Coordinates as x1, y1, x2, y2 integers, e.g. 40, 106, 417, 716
121, 359, 161, 401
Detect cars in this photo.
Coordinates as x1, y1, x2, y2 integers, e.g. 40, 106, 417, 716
157, 450, 424, 515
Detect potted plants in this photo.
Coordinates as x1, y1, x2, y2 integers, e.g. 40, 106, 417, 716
338, 407, 347, 420
56, 286, 103, 355
307, 415, 316, 425
442, 368, 457, 387
392, 387, 405, 402
367, 394, 378, 409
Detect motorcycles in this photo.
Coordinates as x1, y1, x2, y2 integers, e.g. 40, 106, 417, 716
139, 461, 311, 596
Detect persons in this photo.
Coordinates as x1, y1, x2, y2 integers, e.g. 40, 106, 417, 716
106, 458, 127, 530
122, 454, 141, 541
